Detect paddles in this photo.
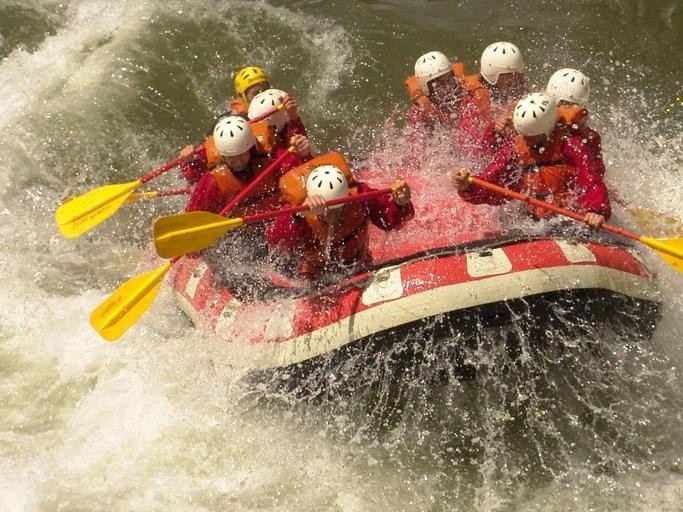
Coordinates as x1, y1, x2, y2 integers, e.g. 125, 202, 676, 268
151, 186, 394, 259
467, 174, 683, 274
616, 192, 683, 238
88, 142, 297, 343
59, 187, 194, 205
53, 101, 283, 241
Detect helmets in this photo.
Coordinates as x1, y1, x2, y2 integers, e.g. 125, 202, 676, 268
513, 92, 556, 137
548, 68, 589, 108
415, 51, 454, 96
248, 89, 290, 132
212, 116, 258, 156
481, 42, 525, 86
235, 67, 268, 94
306, 165, 348, 210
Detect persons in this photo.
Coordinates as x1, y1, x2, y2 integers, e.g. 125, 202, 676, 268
174, 67, 415, 285
404, 40, 612, 232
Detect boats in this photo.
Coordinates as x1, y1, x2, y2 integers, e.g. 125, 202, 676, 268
166, 158, 661, 402
